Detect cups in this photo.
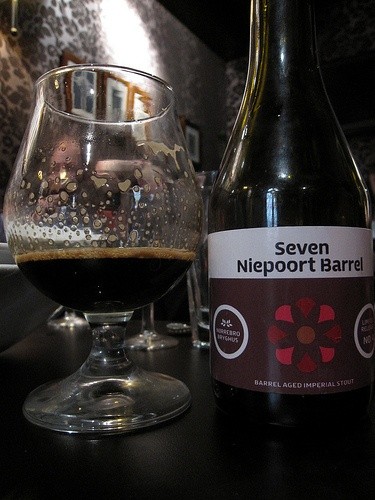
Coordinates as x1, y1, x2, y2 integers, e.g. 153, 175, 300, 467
186, 172, 218, 349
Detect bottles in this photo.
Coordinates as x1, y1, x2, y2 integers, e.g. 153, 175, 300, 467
208, 0, 375, 424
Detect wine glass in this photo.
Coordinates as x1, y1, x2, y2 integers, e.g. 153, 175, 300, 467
123, 304, 179, 349
4, 63, 203, 434
